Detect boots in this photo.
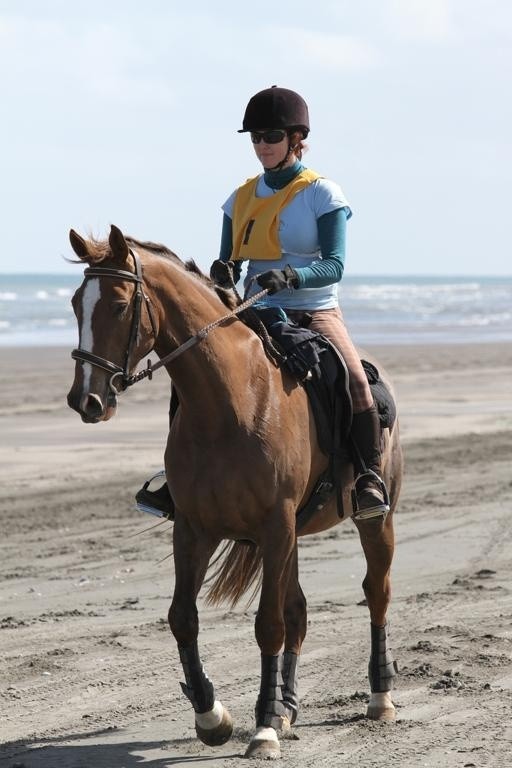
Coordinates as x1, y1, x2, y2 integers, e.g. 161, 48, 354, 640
351, 403, 386, 507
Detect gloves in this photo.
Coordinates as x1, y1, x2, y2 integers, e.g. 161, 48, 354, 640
256, 263, 299, 296
211, 260, 235, 288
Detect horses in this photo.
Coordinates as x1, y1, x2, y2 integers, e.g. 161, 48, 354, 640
61, 224, 404, 760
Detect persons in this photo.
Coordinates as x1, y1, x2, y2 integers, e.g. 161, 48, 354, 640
129, 84, 397, 524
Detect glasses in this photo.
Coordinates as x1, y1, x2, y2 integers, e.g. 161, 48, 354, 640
250, 130, 289, 144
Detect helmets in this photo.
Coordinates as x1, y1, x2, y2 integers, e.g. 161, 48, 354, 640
238, 85, 311, 136
